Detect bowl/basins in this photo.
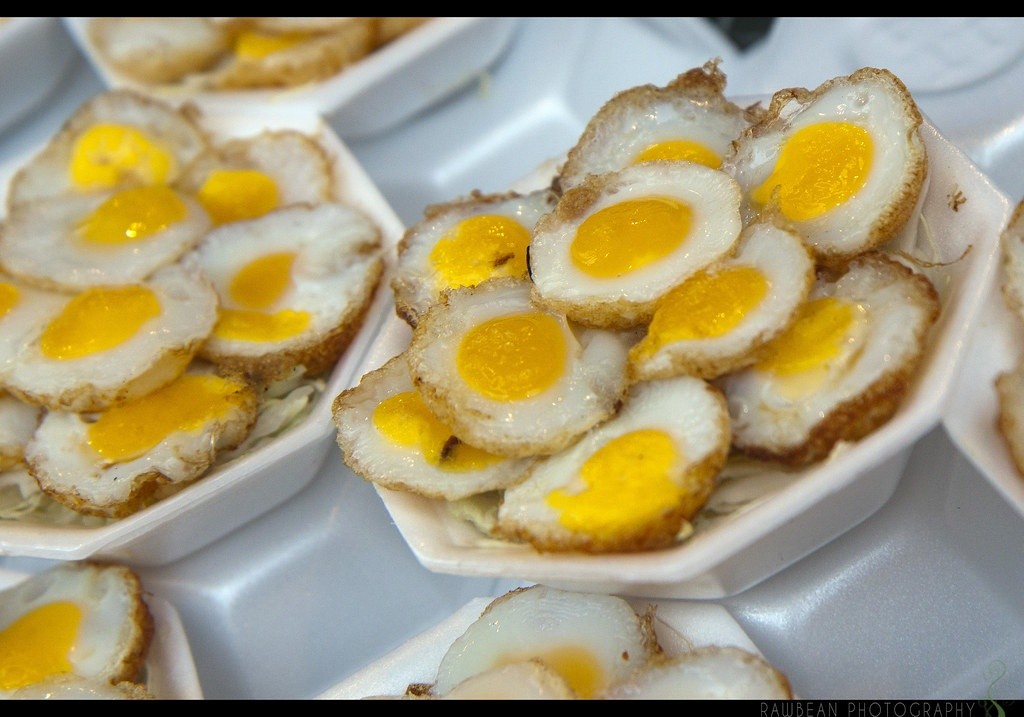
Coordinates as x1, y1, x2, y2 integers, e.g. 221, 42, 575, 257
351, 98, 1010, 601
0, 564, 204, 700
313, 597, 807, 700
58, 17, 516, 143
937, 220, 1024, 514
0, 105, 407, 571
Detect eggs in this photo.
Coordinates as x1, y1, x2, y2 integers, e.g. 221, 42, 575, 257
0, 19, 1024, 699
